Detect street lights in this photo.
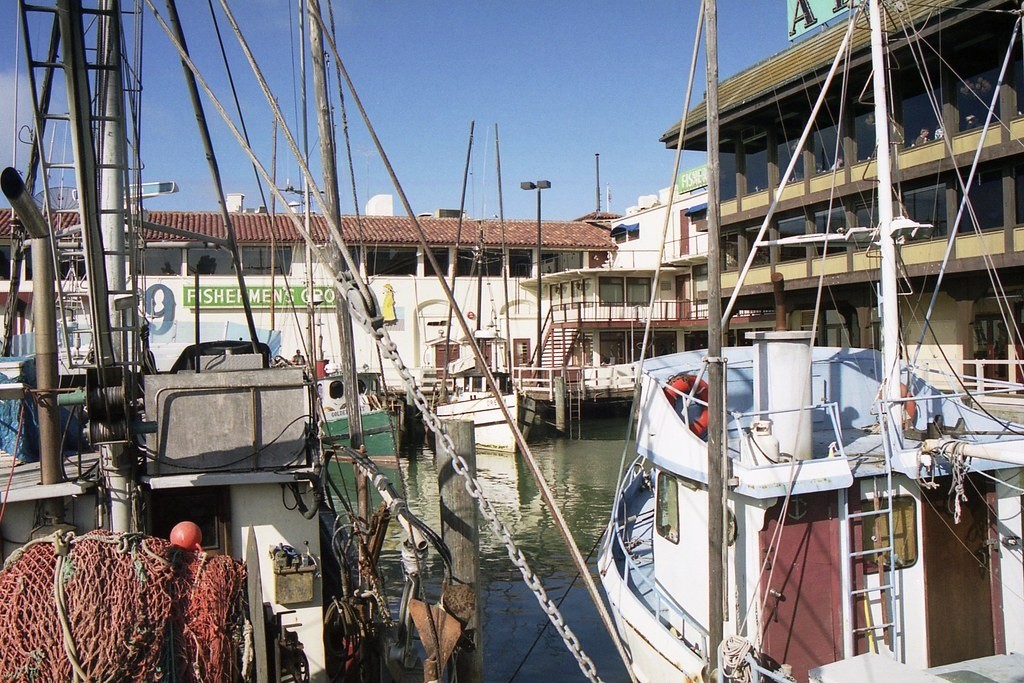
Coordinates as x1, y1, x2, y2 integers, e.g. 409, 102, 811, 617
518, 179, 554, 389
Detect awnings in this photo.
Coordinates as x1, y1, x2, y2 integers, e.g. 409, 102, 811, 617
610, 223, 639, 237
685, 203, 708, 216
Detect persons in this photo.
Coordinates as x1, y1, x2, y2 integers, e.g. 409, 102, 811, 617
830, 156, 843, 170
293, 349, 305, 365
933, 123, 944, 140
959, 114, 982, 132
915, 127, 929, 146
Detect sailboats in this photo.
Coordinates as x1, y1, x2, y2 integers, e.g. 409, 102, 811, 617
0, 0, 428, 683
435, 120, 540, 454
269, 0, 403, 459
594, 0, 1024, 683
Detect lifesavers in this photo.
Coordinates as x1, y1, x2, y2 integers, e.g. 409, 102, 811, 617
661, 375, 711, 437
